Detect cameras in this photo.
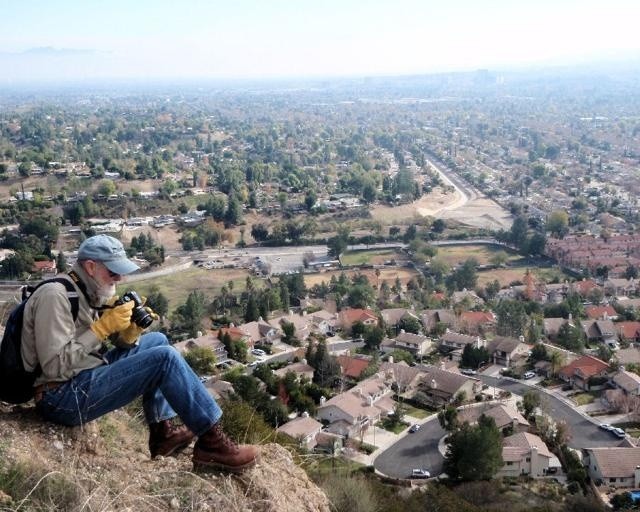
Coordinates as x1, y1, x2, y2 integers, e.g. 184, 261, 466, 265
113, 290, 153, 329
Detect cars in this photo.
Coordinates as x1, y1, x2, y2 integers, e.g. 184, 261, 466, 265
249, 358, 268, 367
199, 375, 208, 383
499, 368, 507, 374
599, 423, 613, 431
252, 348, 265, 355
326, 331, 335, 337
410, 423, 421, 433
216, 359, 237, 370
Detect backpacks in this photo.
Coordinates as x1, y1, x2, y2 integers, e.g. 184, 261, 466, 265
0, 278, 79, 405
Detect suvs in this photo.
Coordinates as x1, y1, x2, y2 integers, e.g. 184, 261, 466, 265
524, 372, 535, 379
411, 468, 431, 479
613, 427, 626, 438
463, 369, 476, 376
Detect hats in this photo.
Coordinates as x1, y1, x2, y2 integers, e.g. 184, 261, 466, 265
78, 234, 140, 275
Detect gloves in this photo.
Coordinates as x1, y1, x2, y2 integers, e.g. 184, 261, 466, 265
120, 296, 158, 344
90, 296, 135, 339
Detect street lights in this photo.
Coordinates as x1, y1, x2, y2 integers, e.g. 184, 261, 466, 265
432, 387, 450, 404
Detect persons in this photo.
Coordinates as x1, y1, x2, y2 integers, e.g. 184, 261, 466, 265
19, 234, 261, 474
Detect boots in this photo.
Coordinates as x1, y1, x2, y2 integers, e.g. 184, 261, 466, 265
192, 422, 260, 472
149, 419, 195, 458
